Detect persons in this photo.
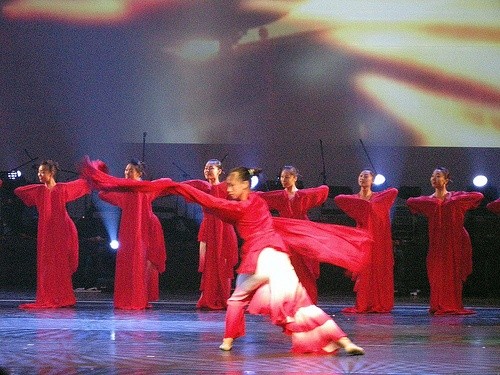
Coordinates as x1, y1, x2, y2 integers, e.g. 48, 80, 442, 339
14, 160, 110, 308
168, 158, 238, 310
98, 159, 172, 311
160, 166, 366, 355
335, 168, 398, 313
407, 166, 484, 314
256, 165, 329, 306
